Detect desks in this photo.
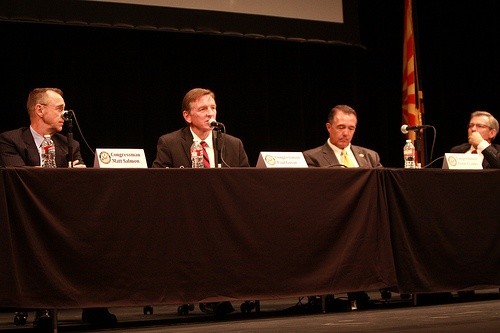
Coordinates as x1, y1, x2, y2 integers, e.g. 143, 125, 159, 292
0, 165, 500, 333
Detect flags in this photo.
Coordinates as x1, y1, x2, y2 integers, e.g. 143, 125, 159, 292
402, 0, 425, 168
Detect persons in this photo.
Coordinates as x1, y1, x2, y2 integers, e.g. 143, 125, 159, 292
152, 89, 250, 313
0, 88, 118, 324
451, 111, 500, 169
303, 105, 383, 300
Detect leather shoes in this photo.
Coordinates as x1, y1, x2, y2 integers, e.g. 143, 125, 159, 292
82, 310, 118, 324
14, 311, 28, 323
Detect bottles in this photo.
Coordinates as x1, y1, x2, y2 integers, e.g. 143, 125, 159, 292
40, 135, 56, 168
404, 139, 416, 168
190, 138, 204, 168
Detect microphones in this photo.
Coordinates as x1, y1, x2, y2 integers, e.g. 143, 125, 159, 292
208, 118, 223, 128
61, 109, 73, 121
401, 124, 430, 134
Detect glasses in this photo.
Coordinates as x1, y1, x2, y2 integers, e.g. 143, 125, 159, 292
468, 122, 492, 129
41, 104, 63, 111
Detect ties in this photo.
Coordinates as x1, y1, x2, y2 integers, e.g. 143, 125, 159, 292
341, 150, 353, 167
471, 147, 477, 154
200, 142, 210, 168
